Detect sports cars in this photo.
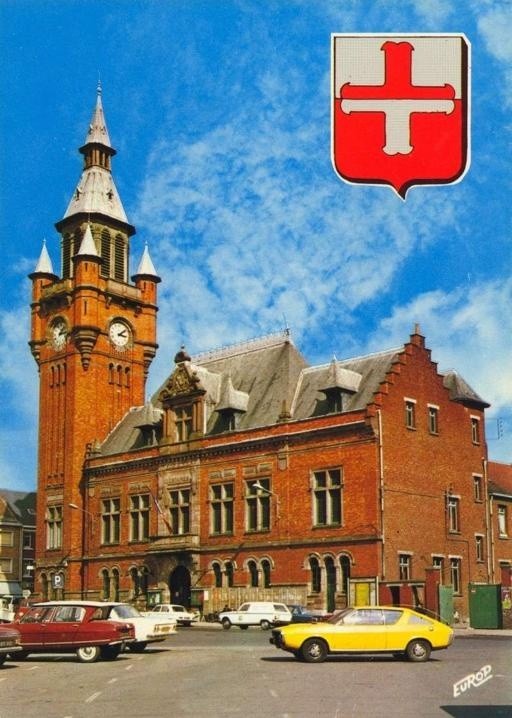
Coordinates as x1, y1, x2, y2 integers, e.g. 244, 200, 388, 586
269, 606, 455, 663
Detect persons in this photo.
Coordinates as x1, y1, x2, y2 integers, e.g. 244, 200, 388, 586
222, 604, 230, 612
414, 601, 427, 615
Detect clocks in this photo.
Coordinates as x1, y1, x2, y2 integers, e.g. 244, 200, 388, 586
50, 319, 68, 347
110, 321, 132, 348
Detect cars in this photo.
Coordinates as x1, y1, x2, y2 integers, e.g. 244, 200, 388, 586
140, 604, 199, 627
0, 594, 177, 670
270, 605, 327, 630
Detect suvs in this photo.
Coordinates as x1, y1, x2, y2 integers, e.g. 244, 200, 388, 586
217, 601, 293, 630
